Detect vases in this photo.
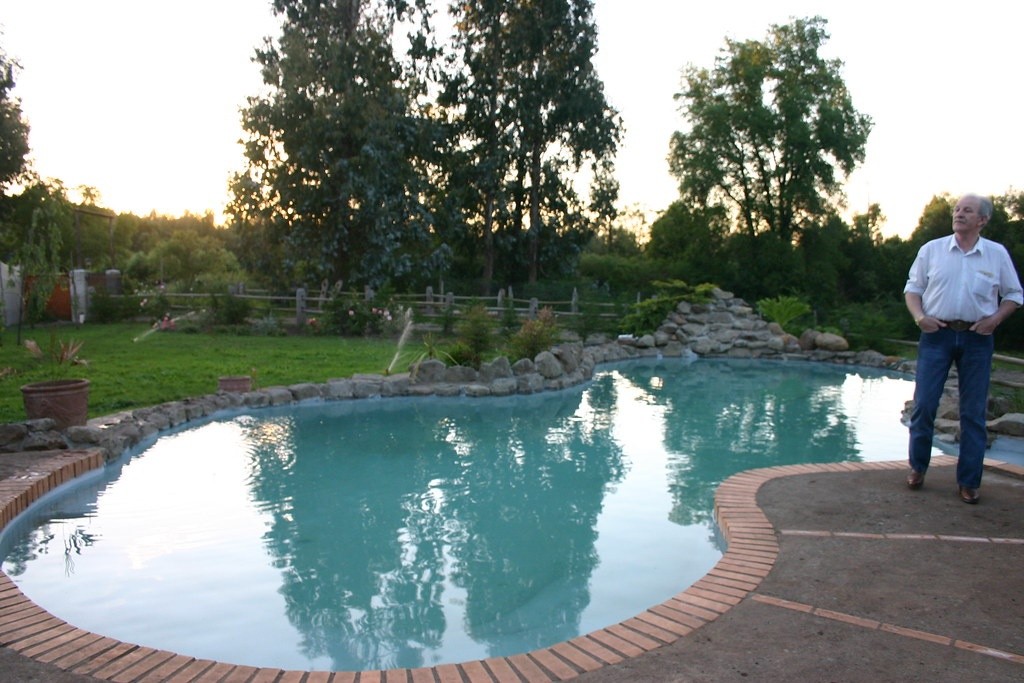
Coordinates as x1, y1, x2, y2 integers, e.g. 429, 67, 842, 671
219, 375, 251, 393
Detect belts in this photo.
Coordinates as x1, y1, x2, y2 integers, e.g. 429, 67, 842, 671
942, 320, 972, 332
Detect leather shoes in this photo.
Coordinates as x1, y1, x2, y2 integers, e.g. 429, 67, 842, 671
906, 467, 925, 487
959, 483, 981, 503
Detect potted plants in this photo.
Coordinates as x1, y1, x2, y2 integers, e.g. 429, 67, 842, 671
19, 336, 89, 430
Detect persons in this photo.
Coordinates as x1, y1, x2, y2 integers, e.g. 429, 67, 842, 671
903, 195, 1024, 504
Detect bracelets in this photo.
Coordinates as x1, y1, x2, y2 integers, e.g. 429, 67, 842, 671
914, 315, 925, 325
